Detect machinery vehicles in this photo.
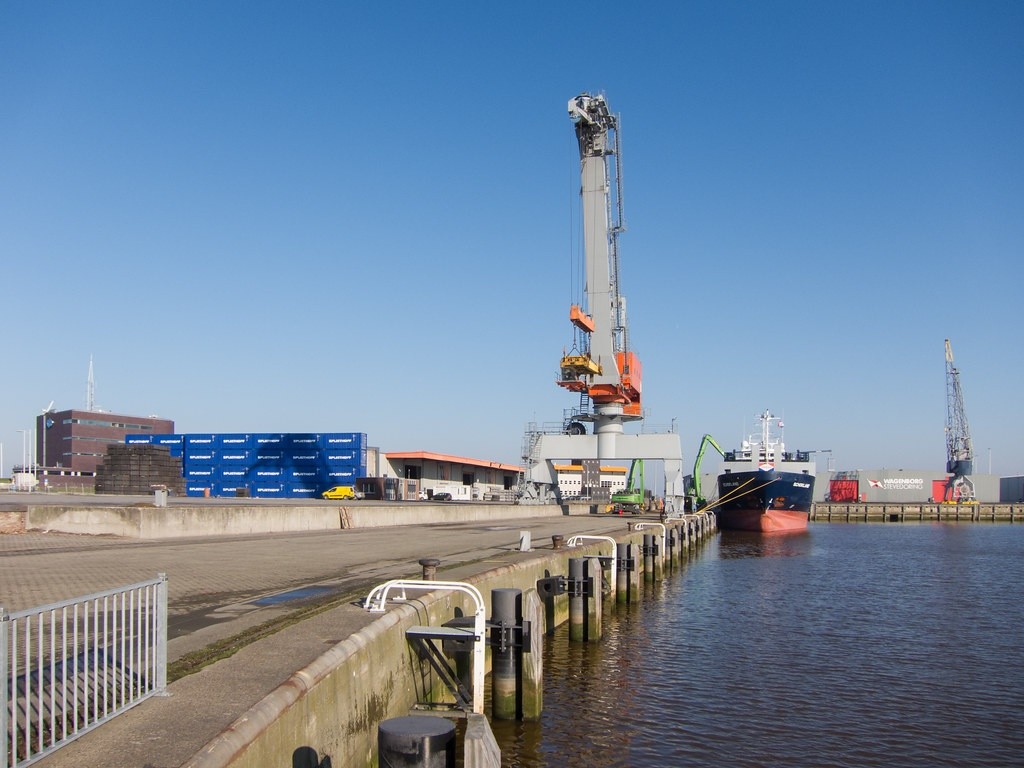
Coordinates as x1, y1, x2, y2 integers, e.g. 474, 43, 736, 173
603, 458, 646, 516
684, 433, 728, 514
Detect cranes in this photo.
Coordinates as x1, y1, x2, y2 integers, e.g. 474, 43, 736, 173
941, 338, 980, 505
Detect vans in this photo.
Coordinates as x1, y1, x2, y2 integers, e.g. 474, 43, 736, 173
322, 486, 355, 500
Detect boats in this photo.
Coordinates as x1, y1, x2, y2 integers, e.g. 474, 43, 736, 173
714, 409, 817, 533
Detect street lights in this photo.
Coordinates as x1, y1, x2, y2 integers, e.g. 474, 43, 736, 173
987, 447, 992, 475
16, 429, 27, 491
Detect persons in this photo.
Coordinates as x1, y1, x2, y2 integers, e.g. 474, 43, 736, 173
619, 508, 622, 517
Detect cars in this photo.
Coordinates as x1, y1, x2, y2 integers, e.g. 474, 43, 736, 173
430, 492, 452, 501
419, 491, 428, 501
354, 490, 366, 500
